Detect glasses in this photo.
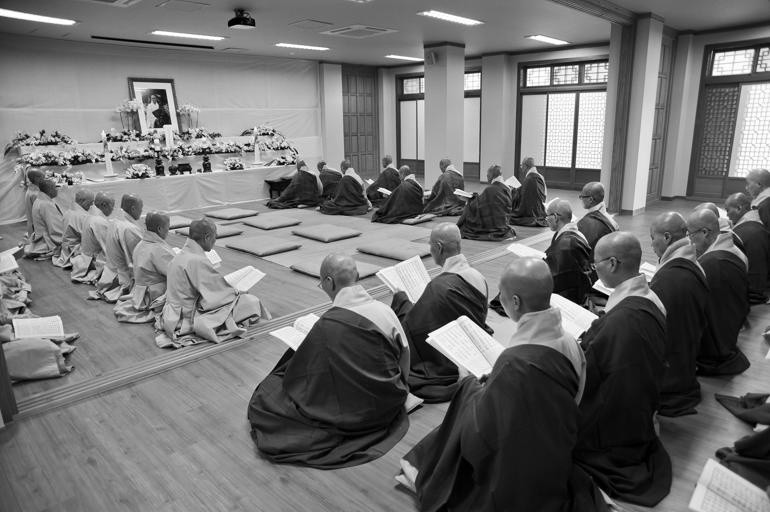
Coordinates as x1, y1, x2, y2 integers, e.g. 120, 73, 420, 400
579, 195, 595, 200
317, 275, 330, 289
544, 213, 562, 221
590, 257, 621, 270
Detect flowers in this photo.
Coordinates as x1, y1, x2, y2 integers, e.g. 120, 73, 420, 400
3, 97, 302, 188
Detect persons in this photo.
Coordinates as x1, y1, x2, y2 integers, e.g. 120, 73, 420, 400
248, 252, 410, 470
578, 231, 672, 508
650, 168, 770, 492
267, 155, 548, 241
0, 249, 80, 379
0, 142, 770, 512
490, 182, 619, 317
395, 257, 620, 512
24, 168, 272, 348
392, 222, 493, 404
146, 94, 169, 129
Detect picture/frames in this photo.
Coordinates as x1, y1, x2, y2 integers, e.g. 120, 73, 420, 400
127, 76, 182, 142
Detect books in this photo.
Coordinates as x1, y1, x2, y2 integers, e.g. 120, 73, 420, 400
453, 189, 473, 198
0, 252, 18, 272
224, 266, 266, 292
376, 255, 431, 304
377, 187, 392, 195
366, 179, 374, 185
510, 244, 547, 259
549, 293, 599, 339
593, 279, 615, 295
205, 250, 221, 268
269, 313, 320, 352
506, 176, 521, 188
689, 460, 770, 511
640, 262, 657, 282
425, 315, 507, 380
12, 316, 64, 339
